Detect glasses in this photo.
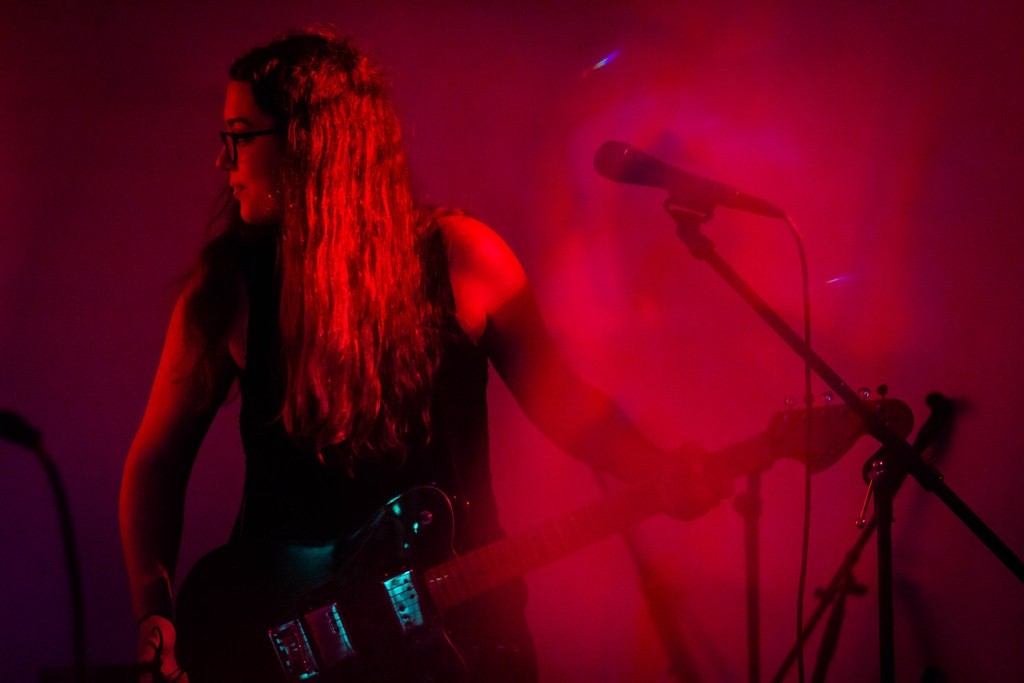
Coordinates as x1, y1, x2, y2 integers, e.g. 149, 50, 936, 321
217, 125, 294, 164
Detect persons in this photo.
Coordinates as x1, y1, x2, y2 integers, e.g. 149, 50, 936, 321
119, 30, 734, 683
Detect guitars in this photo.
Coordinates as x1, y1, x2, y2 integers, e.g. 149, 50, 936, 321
169, 379, 918, 683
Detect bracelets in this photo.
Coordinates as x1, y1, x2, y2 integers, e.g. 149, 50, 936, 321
133, 604, 173, 629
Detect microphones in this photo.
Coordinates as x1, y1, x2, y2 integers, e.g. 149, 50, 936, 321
591, 139, 789, 216
